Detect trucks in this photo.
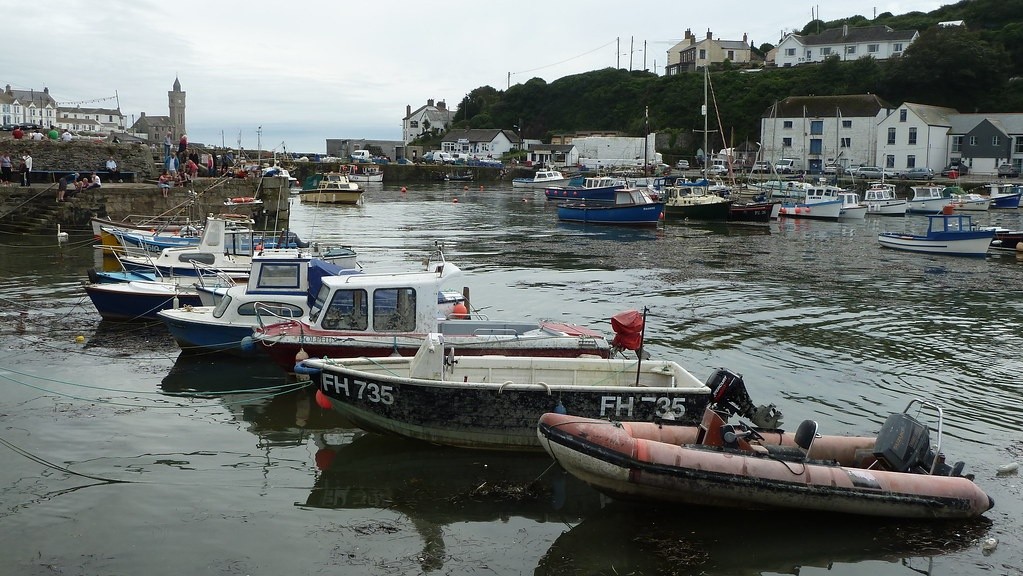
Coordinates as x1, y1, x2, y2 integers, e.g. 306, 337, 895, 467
432, 152, 455, 165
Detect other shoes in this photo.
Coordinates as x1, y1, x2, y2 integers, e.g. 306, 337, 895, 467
109, 179, 112, 183
58, 198, 65, 201
118, 179, 123, 183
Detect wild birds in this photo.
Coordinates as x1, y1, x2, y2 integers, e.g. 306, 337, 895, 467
57, 224, 69, 237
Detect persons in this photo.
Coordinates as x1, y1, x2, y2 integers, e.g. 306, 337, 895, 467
12, 124, 73, 141
111, 135, 120, 143
207, 148, 234, 178
263, 169, 280, 177
175, 133, 187, 155
0, 150, 13, 185
58, 172, 101, 202
106, 156, 123, 183
19, 150, 33, 187
158, 148, 199, 197
163, 131, 172, 156
473, 153, 493, 159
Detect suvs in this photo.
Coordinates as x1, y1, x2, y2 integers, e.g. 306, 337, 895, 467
997, 163, 1019, 177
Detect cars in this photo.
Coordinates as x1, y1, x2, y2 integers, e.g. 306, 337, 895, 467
824, 164, 935, 179
641, 158, 803, 176
941, 161, 969, 177
351, 150, 372, 160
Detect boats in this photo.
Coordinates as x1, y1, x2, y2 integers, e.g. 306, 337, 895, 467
339, 165, 385, 183
298, 172, 365, 202
535, 396, 995, 525
262, 165, 298, 189
877, 213, 996, 255
513, 168, 583, 189
242, 240, 652, 378
546, 172, 1023, 227
956, 222, 1023, 251
83, 196, 466, 357
294, 305, 785, 452
442, 170, 474, 182
